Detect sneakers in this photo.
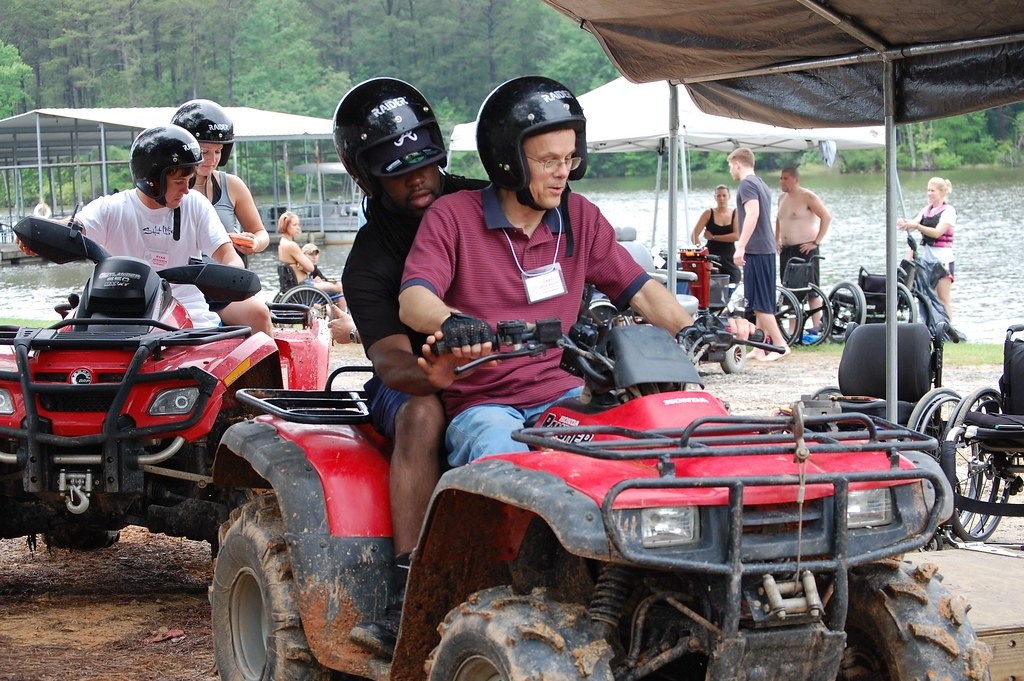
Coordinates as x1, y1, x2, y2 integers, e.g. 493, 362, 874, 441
349, 610, 406, 660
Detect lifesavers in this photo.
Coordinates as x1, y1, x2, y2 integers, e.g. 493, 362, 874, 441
34, 204, 51, 219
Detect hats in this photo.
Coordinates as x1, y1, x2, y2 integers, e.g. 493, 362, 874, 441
369, 123, 447, 178
302, 243, 319, 255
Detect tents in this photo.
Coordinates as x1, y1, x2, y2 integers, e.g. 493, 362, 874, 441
449, 62, 895, 298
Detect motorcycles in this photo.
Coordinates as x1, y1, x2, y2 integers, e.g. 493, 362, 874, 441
822, 260, 932, 343
770, 256, 833, 348
0, 216, 333, 558
586, 226, 745, 374
204, 313, 996, 681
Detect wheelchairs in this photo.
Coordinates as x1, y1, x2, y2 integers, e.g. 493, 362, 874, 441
804, 319, 1024, 541
270, 265, 333, 333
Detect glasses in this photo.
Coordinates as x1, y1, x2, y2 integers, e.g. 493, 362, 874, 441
526, 154, 582, 174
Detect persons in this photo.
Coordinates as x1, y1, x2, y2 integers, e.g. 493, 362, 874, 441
278, 211, 346, 315
18, 97, 271, 341
690, 148, 831, 364
332, 76, 501, 663
396, 76, 753, 471
898, 177, 958, 327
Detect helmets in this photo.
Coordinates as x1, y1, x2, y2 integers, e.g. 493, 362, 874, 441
474, 75, 587, 192
171, 98, 235, 166
129, 123, 204, 200
332, 76, 447, 200
